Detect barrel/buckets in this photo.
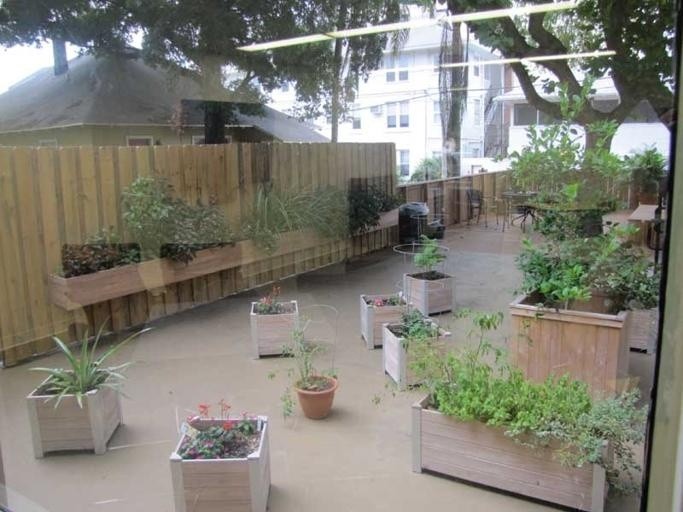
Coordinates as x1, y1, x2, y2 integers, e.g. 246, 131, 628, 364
399, 201, 429, 244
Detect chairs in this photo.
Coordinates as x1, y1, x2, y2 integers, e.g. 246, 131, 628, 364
466, 189, 498, 227
502, 194, 535, 233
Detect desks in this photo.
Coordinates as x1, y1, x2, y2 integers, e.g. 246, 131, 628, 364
501, 192, 538, 229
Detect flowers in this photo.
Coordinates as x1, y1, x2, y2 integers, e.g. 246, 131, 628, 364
366, 294, 400, 307
259, 286, 281, 314
181, 399, 256, 459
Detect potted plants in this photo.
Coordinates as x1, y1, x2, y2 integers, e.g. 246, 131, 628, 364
407, 307, 647, 512
509, 75, 663, 401
269, 329, 338, 420
383, 309, 452, 393
623, 148, 666, 205
403, 234, 455, 316
28, 314, 155, 458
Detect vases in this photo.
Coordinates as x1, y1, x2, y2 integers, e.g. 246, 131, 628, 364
360, 292, 414, 351
250, 300, 299, 360
170, 414, 270, 510
